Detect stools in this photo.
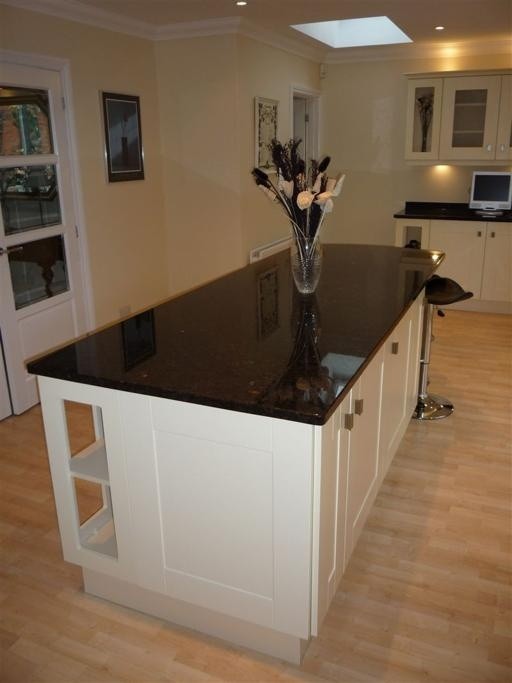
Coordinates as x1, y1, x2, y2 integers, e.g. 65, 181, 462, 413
412, 275, 475, 422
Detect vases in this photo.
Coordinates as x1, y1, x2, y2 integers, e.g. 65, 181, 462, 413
289, 233, 324, 296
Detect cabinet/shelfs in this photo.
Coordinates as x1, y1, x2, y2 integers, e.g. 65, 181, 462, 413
437, 74, 511, 161
310, 344, 385, 637
429, 218, 512, 303
403, 78, 443, 162
395, 217, 428, 248
379, 285, 427, 484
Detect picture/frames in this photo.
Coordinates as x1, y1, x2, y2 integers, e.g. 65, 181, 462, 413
120, 307, 157, 372
252, 94, 280, 175
97, 87, 146, 183
255, 265, 281, 343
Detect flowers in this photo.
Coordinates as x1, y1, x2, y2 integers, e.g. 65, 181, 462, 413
256, 303, 335, 408
250, 136, 345, 281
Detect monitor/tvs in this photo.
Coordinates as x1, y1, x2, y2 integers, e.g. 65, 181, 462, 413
469, 170, 512, 218
